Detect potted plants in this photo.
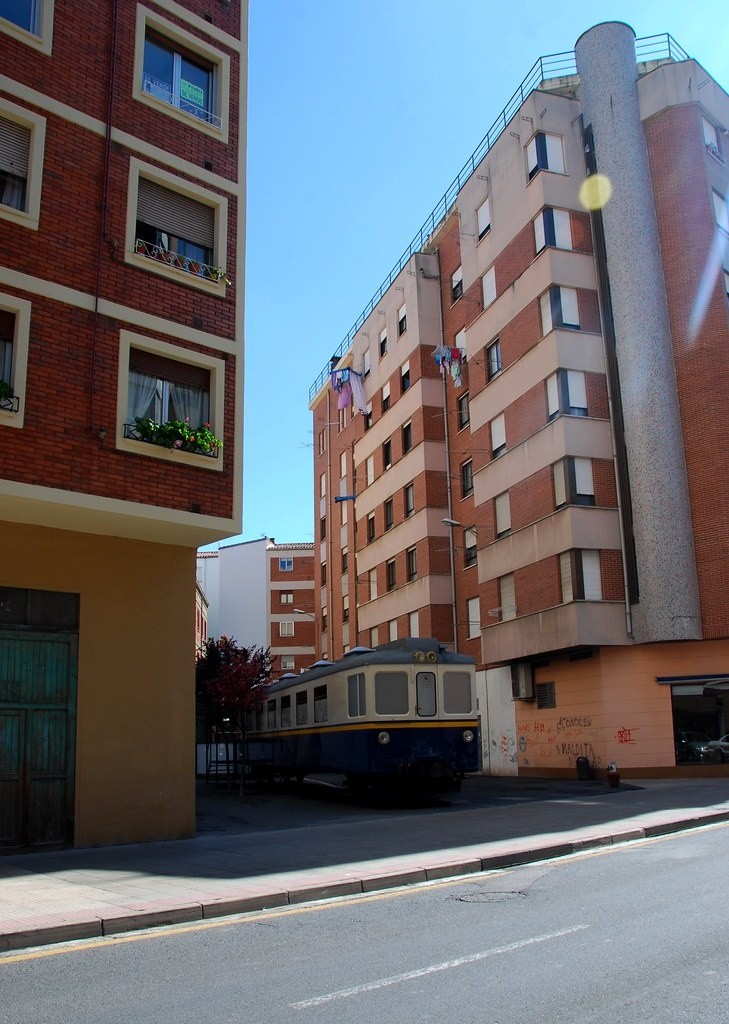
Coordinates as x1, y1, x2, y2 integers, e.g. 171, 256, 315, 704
128, 415, 159, 440
158, 240, 168, 261
189, 260, 202, 273
174, 255, 186, 266
0, 379, 16, 409
204, 264, 231, 287
137, 241, 146, 254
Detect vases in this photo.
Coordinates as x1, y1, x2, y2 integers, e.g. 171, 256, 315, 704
194, 446, 215, 457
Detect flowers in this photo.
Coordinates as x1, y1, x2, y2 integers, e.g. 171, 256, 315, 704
156, 416, 223, 455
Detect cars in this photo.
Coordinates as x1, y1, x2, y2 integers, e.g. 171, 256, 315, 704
673, 731, 729, 764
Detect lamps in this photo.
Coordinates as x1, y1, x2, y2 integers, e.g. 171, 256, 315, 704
441, 518, 478, 539
293, 608, 320, 624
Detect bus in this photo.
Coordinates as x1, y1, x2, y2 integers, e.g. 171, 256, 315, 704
244, 635, 483, 804
